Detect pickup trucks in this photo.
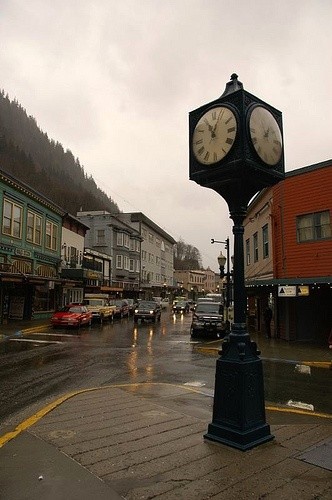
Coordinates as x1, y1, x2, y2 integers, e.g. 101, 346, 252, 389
82, 298, 117, 325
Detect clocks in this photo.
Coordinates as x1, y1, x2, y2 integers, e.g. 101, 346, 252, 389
244, 102, 283, 168
190, 102, 242, 169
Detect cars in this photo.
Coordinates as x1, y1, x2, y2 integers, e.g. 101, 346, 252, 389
51, 304, 92, 329
134, 300, 161, 322
172, 293, 222, 314
108, 297, 169, 318
190, 302, 224, 336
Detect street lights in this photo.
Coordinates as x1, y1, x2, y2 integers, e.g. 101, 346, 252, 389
211, 238, 230, 332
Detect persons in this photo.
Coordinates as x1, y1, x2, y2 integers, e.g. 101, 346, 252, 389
264, 304, 272, 338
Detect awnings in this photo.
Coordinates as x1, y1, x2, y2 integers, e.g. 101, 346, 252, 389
244, 257, 274, 281
